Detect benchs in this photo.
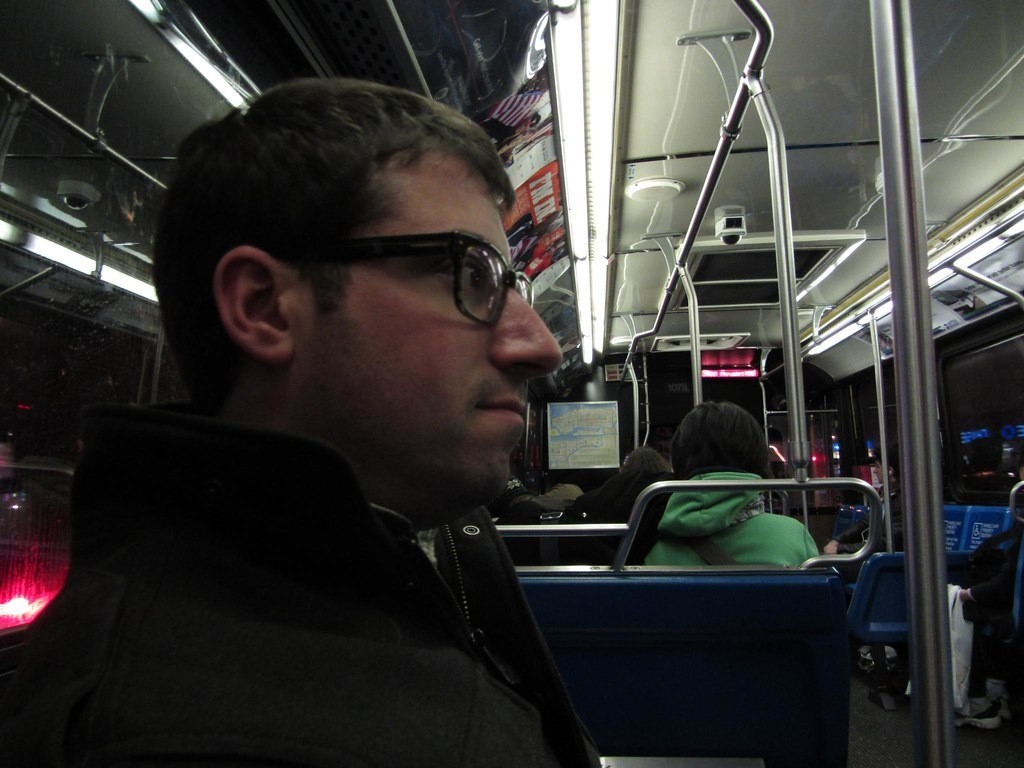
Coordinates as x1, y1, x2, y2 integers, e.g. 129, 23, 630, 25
490, 503, 1024, 768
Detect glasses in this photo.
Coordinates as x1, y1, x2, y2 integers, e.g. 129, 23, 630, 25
261, 229, 533, 325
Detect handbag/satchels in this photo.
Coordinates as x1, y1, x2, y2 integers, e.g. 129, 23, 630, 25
904, 585, 975, 708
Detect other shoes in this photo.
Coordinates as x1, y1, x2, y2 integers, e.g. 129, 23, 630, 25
952, 704, 1001, 728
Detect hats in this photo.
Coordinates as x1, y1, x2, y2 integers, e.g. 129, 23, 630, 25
485, 474, 527, 512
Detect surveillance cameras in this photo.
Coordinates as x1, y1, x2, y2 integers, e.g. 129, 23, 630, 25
714, 216, 747, 245
57, 181, 99, 209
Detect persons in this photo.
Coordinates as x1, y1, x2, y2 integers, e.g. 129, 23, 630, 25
490, 443, 674, 565
639, 399, 820, 572
0, 78, 604, 768
945, 442, 1024, 729
822, 443, 904, 585
480, 105, 543, 169
